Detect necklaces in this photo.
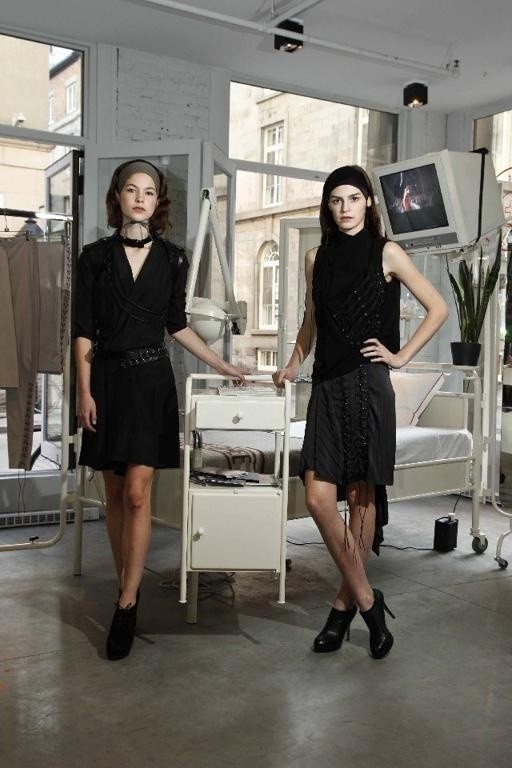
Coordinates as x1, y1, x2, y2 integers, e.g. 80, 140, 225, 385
116, 233, 155, 249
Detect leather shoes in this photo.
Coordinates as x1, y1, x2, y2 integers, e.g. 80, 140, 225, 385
106, 588, 140, 660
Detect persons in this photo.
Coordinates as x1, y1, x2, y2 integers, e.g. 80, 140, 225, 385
269, 162, 452, 659
388, 175, 435, 214
60, 158, 255, 663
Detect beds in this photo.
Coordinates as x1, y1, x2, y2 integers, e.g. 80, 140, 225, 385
70, 357, 488, 625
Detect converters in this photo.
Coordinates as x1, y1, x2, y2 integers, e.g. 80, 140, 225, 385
433, 515, 458, 553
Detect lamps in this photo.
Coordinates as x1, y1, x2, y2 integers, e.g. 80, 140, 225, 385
273, 19, 305, 52
403, 83, 428, 108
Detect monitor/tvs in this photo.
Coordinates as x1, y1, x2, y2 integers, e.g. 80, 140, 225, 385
371, 150, 506, 256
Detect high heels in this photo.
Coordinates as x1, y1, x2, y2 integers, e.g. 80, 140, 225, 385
314, 605, 357, 652
359, 589, 395, 659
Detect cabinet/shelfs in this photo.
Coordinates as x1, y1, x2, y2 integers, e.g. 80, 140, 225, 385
179, 374, 293, 604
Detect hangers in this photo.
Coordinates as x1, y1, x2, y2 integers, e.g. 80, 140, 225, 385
0, 206, 70, 247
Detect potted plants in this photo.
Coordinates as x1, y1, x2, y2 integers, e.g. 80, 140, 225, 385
445, 227, 504, 366
